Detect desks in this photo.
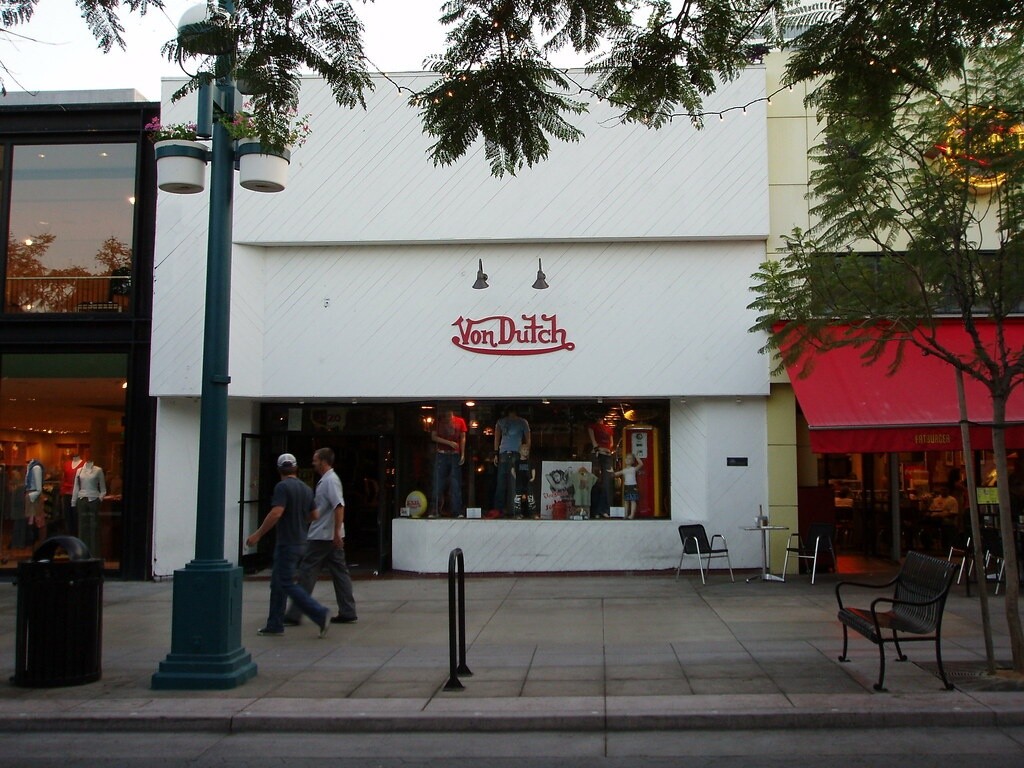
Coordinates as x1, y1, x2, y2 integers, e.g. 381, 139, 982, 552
834, 497, 906, 553
739, 525, 790, 582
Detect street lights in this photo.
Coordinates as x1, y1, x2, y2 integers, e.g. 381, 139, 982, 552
150, 7, 292, 694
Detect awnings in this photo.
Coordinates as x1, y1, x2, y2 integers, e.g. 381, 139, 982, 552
770, 319, 1024, 450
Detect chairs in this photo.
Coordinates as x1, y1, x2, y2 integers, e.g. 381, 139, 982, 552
782, 523, 837, 586
943, 534, 1006, 595
676, 524, 736, 587
834, 503, 963, 555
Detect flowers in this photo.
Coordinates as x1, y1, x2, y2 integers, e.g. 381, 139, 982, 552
143, 117, 198, 144
921, 493, 934, 507
217, 92, 314, 158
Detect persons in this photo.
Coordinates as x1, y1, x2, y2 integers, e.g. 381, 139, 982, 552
60, 455, 85, 537
947, 467, 965, 505
611, 453, 644, 519
511, 444, 540, 519
25, 458, 43, 559
71, 460, 106, 556
494, 411, 531, 516
588, 419, 614, 517
920, 487, 959, 555
283, 447, 357, 624
429, 410, 467, 518
246, 454, 332, 638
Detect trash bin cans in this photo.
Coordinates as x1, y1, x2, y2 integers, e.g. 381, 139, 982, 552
14, 535, 104, 687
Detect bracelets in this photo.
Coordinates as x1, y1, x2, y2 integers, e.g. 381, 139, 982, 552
494, 451, 498, 455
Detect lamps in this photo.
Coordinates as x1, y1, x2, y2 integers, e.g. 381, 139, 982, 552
532, 258, 550, 290
120, 378, 127, 388
542, 398, 550, 404
680, 396, 686, 403
472, 258, 489, 289
735, 395, 743, 403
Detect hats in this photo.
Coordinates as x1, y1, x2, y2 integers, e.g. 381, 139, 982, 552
278, 454, 297, 468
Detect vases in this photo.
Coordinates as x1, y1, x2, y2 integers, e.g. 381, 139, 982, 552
920, 498, 931, 511
236, 136, 292, 194
153, 140, 208, 194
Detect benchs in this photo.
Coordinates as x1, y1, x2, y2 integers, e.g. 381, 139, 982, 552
835, 551, 960, 693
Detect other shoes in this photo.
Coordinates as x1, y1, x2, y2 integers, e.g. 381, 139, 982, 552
317, 609, 332, 639
257, 624, 286, 636
330, 611, 357, 623
280, 615, 300, 626
